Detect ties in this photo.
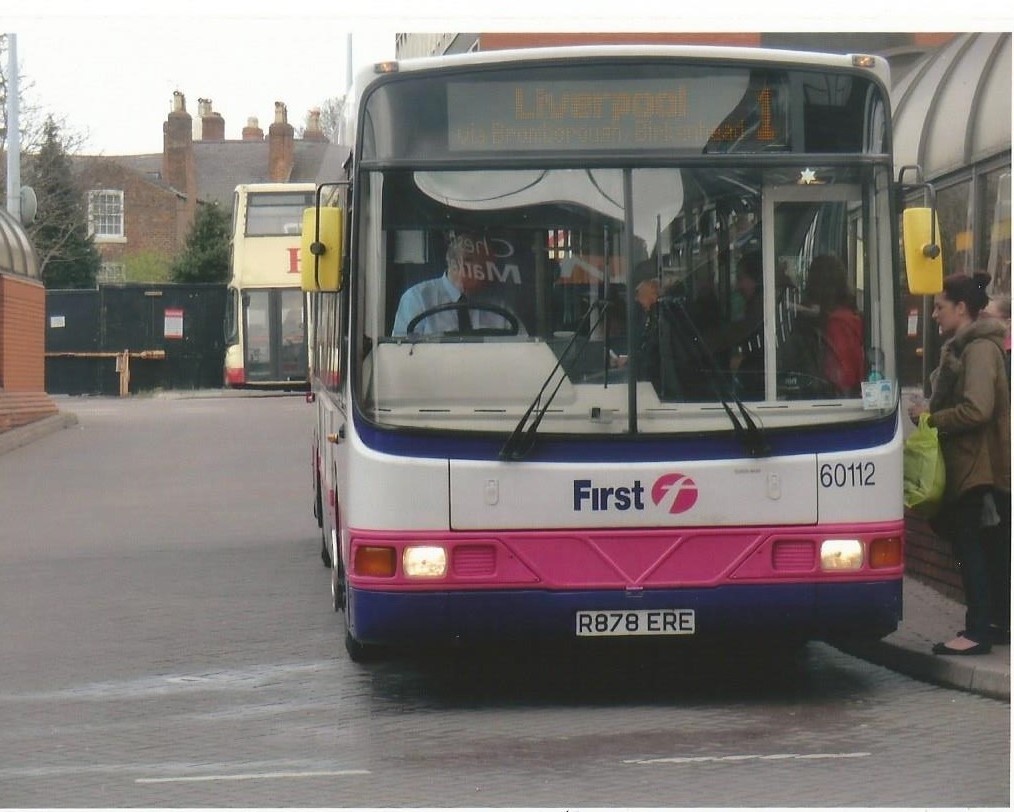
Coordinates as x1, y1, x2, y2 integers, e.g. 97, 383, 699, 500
457, 293, 472, 333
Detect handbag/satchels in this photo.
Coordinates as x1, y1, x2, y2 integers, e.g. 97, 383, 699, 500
905, 412, 947, 520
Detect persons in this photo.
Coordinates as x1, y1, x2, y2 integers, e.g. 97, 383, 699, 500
907, 273, 1012, 653
392, 233, 527, 336
731, 251, 801, 398
801, 255, 864, 394
611, 259, 733, 400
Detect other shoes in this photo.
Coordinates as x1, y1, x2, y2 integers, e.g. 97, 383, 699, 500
955, 630, 1010, 643
931, 638, 990, 655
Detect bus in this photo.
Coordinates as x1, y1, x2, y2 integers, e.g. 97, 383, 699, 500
298, 40, 945, 667
221, 180, 338, 394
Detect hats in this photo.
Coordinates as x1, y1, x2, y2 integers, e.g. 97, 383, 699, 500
809, 254, 855, 281
